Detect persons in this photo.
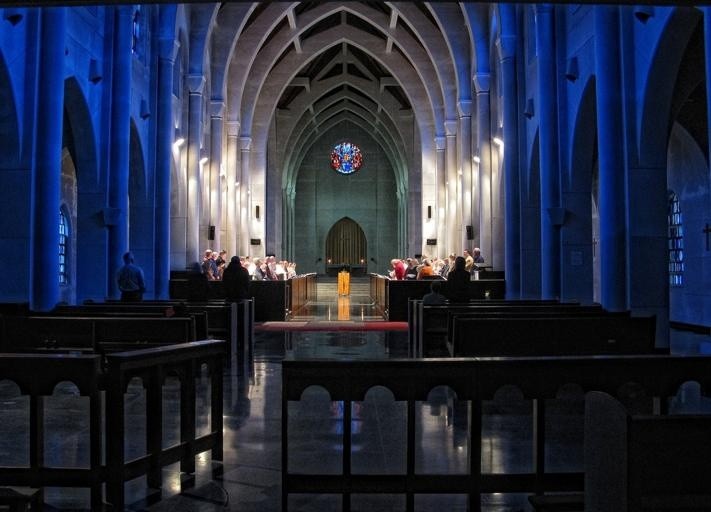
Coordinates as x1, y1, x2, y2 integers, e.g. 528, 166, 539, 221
445, 254, 475, 302
422, 281, 450, 305
224, 253, 249, 298
115, 248, 147, 299
388, 240, 488, 277
200, 243, 297, 286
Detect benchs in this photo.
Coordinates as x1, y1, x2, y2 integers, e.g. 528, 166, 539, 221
398, 295, 662, 360
29, 294, 255, 364
